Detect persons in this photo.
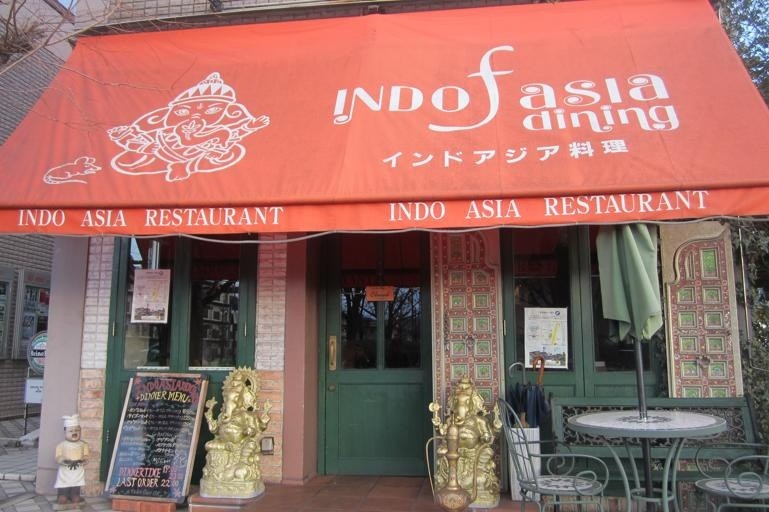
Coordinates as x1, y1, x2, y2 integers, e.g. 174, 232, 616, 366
55, 414, 91, 504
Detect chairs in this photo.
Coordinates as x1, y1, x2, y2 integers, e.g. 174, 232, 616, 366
497, 397, 609, 512
695, 442, 769, 512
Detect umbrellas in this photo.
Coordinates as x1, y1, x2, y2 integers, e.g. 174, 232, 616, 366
596, 223, 664, 511
527, 355, 554, 427
507, 361, 527, 423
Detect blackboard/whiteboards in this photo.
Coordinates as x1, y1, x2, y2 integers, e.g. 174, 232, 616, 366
103, 372, 209, 504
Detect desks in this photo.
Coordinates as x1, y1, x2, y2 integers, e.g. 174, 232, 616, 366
567, 410, 727, 512
